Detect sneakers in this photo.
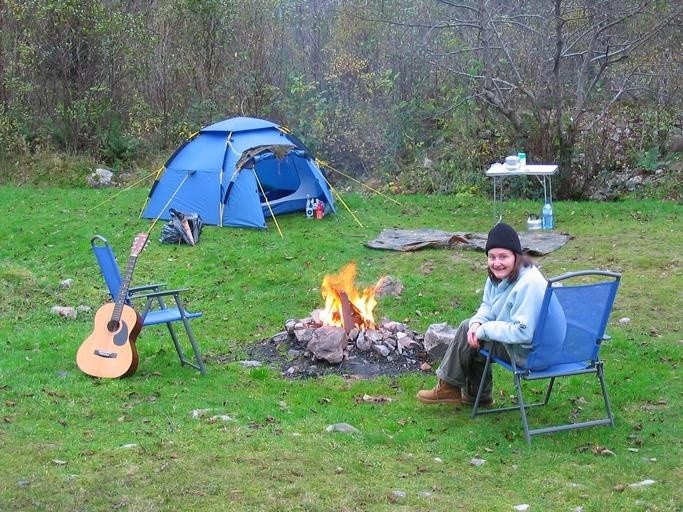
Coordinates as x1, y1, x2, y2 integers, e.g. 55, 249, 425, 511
461, 393, 495, 406
417, 379, 462, 404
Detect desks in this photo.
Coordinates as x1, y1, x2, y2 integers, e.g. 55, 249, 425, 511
487, 163, 559, 227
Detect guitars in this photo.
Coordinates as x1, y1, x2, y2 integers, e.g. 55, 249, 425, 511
77, 232, 150, 378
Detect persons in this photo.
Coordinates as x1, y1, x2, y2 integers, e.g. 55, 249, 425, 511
415, 222, 567, 409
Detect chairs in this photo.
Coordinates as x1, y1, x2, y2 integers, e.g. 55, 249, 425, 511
90, 235, 206, 374
470, 269, 622, 446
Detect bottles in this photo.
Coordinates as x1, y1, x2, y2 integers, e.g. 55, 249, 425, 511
320, 201, 325, 216
306, 195, 313, 219
542, 201, 552, 230
317, 204, 322, 219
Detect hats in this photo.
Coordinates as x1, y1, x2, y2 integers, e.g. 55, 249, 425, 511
485, 223, 522, 256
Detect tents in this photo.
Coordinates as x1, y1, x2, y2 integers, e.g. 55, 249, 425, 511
138, 117, 337, 231
